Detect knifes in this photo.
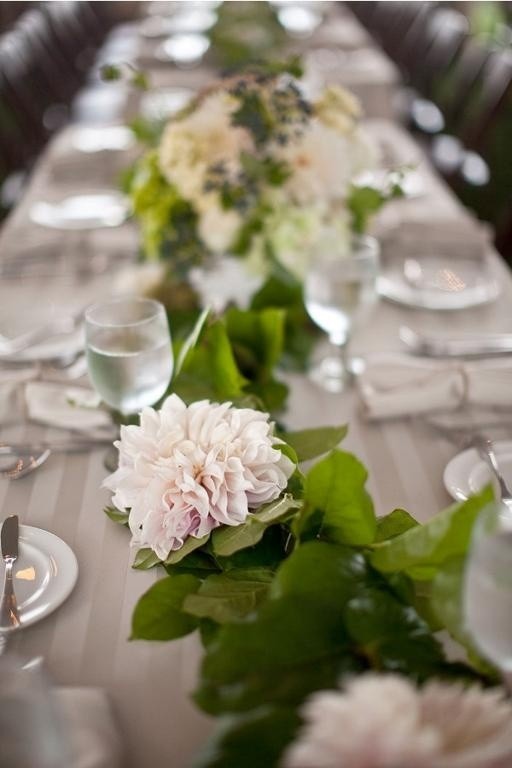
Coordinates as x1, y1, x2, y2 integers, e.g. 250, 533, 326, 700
1, 513, 21, 633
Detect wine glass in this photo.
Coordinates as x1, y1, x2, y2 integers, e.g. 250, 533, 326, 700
301, 232, 381, 395
86, 298, 175, 471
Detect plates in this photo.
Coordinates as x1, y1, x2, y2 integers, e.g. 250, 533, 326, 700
373, 259, 501, 313
2, 520, 78, 640
444, 436, 512, 506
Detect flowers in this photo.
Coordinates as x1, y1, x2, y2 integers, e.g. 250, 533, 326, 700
149, 68, 377, 312
274, 659, 510, 766
105, 391, 296, 561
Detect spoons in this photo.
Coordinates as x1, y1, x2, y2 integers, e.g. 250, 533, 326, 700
0, 438, 51, 478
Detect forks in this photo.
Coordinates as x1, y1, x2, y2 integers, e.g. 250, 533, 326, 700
397, 324, 511, 361
